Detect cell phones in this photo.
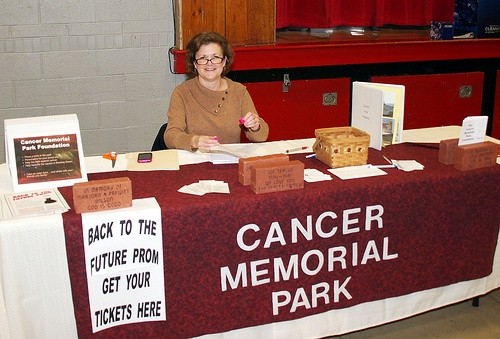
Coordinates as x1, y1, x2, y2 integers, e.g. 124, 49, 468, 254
137, 153, 152, 163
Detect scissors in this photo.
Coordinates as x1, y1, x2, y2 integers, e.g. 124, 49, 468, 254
102, 152, 117, 167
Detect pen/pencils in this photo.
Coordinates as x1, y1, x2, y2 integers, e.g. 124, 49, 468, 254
286, 146, 309, 153
374, 165, 396, 169
306, 153, 317, 159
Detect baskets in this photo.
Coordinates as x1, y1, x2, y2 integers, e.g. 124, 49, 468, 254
314, 127, 369, 167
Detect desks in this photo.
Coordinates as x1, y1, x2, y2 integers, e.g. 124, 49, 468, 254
0, 125, 500, 339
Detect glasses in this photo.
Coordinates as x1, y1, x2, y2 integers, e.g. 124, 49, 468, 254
194, 55, 225, 65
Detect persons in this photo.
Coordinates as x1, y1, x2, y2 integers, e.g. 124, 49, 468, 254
163, 32, 268, 153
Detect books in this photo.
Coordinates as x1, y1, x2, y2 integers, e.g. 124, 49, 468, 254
351, 82, 404, 151
